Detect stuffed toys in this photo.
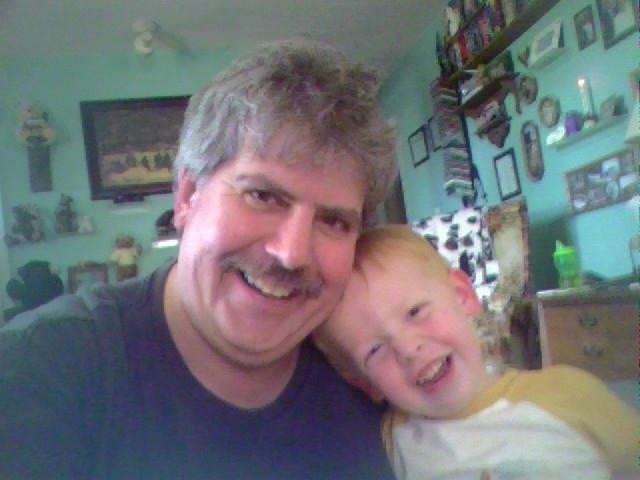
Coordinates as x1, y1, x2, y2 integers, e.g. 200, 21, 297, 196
3, 203, 43, 242
12, 97, 55, 147
4, 259, 62, 322
109, 234, 143, 280
55, 191, 73, 232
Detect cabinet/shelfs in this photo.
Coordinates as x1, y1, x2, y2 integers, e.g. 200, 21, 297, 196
435, 0, 568, 135
538, 292, 640, 383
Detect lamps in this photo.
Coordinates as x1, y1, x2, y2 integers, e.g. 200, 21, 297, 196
147, 209, 180, 250
130, 24, 161, 57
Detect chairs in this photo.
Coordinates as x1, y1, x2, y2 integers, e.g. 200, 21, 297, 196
480, 197, 531, 367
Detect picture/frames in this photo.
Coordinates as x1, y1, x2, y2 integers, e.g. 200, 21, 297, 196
493, 148, 521, 200
564, 144, 640, 216
594, 1, 634, 49
428, 115, 443, 149
527, 20, 560, 65
408, 126, 430, 168
79, 95, 194, 199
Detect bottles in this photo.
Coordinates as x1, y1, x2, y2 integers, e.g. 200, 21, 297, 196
554, 240, 581, 289
564, 109, 578, 137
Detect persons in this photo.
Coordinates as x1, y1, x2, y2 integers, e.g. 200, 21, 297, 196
0, 44, 394, 479
310, 223, 639, 480
109, 152, 172, 173
